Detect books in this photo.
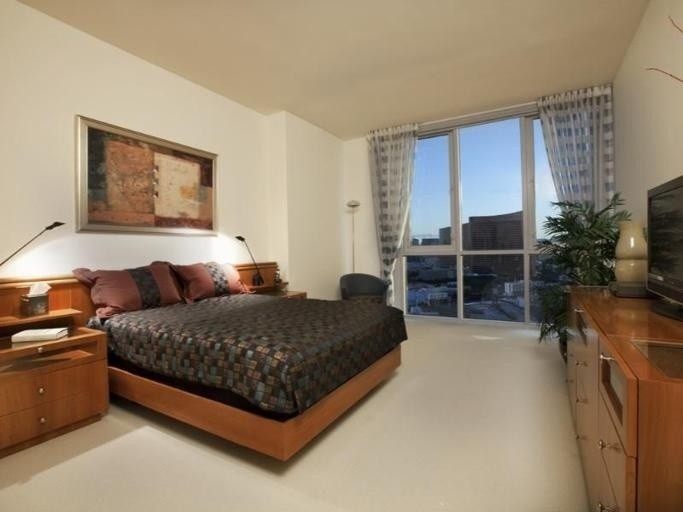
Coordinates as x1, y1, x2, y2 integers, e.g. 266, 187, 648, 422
10, 326, 69, 342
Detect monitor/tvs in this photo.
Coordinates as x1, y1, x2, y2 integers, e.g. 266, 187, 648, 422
645, 174, 683, 320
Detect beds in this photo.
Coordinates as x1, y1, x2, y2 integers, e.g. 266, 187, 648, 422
87, 292, 402, 462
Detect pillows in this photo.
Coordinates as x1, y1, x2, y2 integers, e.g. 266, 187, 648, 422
171, 263, 257, 304
74, 262, 194, 317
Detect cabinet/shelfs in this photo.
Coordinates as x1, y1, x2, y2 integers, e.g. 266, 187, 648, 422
243, 283, 289, 290
0, 306, 108, 459
566, 288, 683, 512
264, 291, 308, 300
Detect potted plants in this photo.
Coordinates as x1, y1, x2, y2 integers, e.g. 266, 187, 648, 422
535, 192, 630, 362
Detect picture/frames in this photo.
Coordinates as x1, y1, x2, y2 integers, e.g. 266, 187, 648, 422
75, 116, 219, 238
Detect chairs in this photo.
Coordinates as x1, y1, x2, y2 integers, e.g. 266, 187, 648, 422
340, 274, 388, 305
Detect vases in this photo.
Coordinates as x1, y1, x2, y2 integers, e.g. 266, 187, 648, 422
615, 221, 648, 283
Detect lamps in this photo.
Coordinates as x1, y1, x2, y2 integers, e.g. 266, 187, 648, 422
235, 236, 264, 285
0, 221, 66, 268
347, 199, 360, 273
